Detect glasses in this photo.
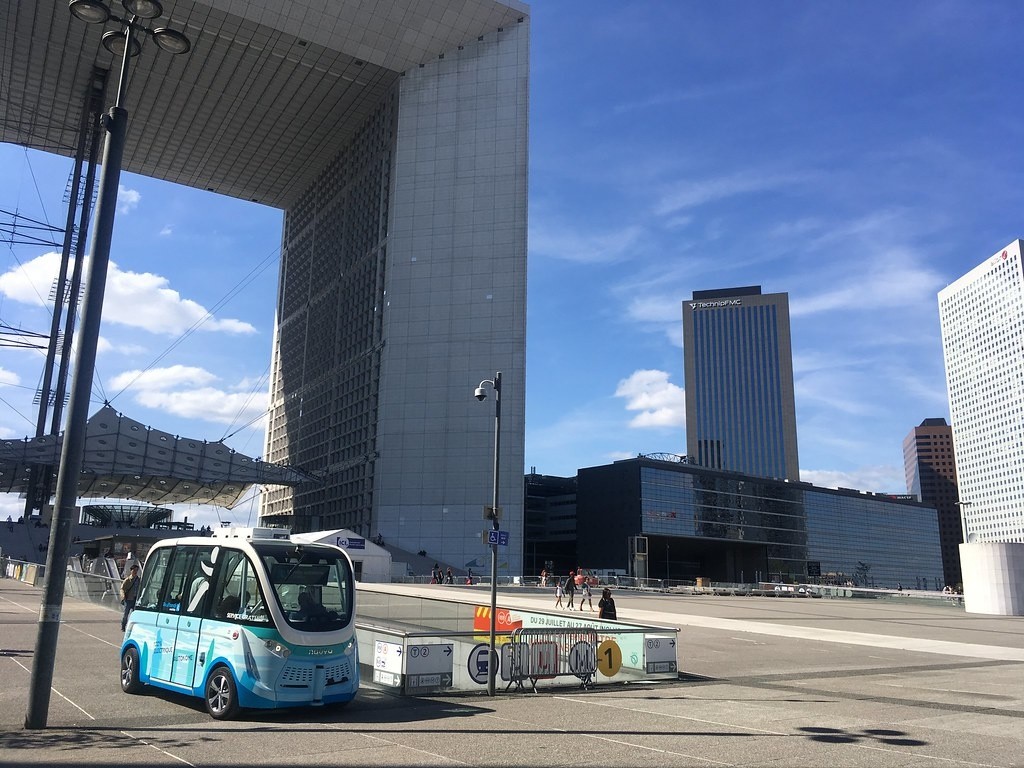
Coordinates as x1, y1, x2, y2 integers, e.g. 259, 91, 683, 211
606, 592, 612, 595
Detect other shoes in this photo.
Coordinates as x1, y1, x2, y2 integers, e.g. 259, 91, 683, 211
567, 607, 575, 611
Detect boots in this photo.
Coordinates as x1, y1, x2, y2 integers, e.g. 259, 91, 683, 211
590, 606, 594, 612
579, 606, 583, 610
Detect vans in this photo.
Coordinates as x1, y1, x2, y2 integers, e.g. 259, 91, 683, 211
121, 527, 359, 720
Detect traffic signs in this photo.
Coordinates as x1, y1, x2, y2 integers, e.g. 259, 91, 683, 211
372, 639, 404, 687
405, 643, 454, 689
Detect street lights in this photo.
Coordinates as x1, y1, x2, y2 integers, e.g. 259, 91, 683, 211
476, 373, 503, 698
26, 1, 191, 735
955, 502, 972, 542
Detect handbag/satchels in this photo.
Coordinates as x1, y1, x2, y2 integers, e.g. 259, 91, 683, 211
587, 592, 592, 597
120, 601, 125, 605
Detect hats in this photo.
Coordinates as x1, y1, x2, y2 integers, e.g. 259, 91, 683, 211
570, 571, 575, 575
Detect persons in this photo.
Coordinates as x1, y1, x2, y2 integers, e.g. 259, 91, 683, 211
541, 570, 547, 587
201, 525, 211, 532
39, 543, 43, 551
74, 535, 80, 542
578, 565, 582, 575
793, 579, 799, 597
554, 572, 594, 612
118, 564, 140, 631
92, 516, 138, 528
220, 590, 251, 618
432, 567, 454, 584
292, 592, 328, 621
434, 562, 440, 569
598, 588, 617, 621
941, 583, 962, 606
18, 516, 25, 524
156, 576, 182, 611
468, 568, 474, 584
